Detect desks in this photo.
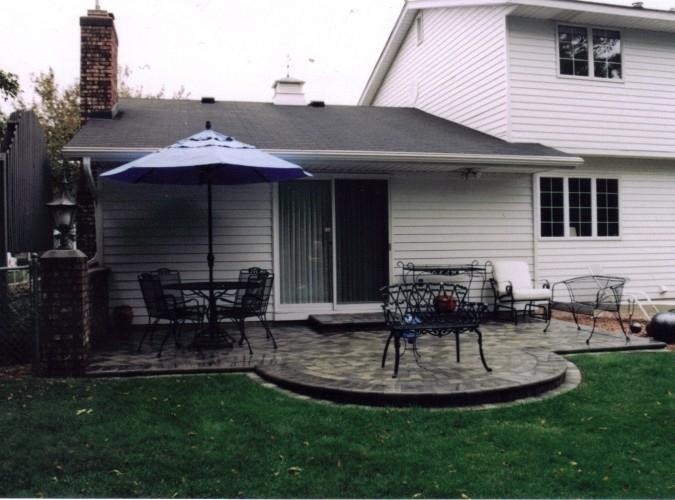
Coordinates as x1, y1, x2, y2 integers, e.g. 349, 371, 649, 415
397, 259, 486, 304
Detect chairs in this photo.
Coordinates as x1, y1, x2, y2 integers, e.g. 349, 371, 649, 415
489, 260, 553, 324
589, 265, 659, 321
136, 265, 279, 356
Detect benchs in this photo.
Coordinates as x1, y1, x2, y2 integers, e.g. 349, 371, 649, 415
543, 274, 630, 345
380, 285, 492, 378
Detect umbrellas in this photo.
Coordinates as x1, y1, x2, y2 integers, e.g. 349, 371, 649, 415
99, 121, 315, 282
